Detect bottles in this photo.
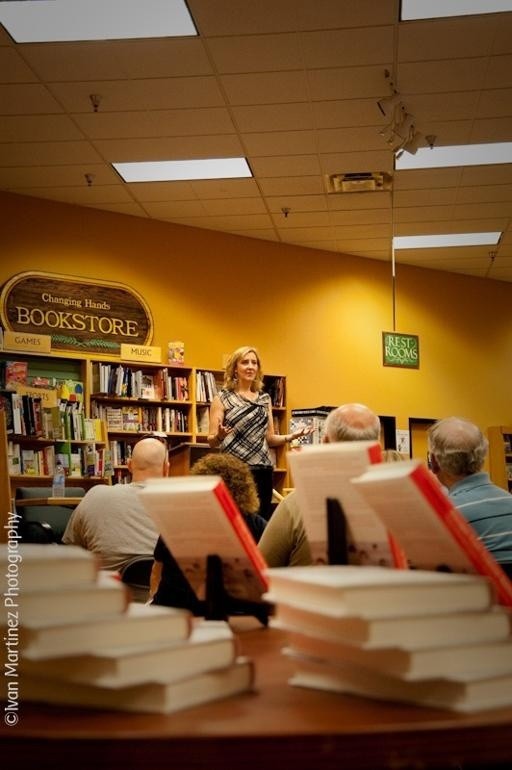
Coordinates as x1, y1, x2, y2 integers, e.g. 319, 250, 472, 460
52, 459, 65, 497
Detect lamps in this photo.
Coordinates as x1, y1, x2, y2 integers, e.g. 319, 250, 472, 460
377, 77, 421, 156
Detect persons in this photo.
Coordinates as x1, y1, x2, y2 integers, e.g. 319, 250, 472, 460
138, 454, 270, 626
425, 415, 512, 567
255, 402, 405, 568
59, 434, 172, 568
205, 346, 319, 504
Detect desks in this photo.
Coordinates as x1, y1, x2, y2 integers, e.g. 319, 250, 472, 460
13, 595, 510, 769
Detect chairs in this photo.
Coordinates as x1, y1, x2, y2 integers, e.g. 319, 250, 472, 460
12, 484, 86, 543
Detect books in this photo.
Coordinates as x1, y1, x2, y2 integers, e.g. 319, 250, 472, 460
137, 474, 270, 601
285, 440, 408, 570
0, 534, 256, 716
4, 359, 284, 485
349, 459, 512, 606
256, 560, 512, 717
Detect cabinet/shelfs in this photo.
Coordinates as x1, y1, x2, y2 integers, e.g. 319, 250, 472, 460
2, 347, 290, 536
487, 424, 511, 494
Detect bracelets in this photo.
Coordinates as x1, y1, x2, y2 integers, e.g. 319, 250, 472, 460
285, 434, 290, 444
